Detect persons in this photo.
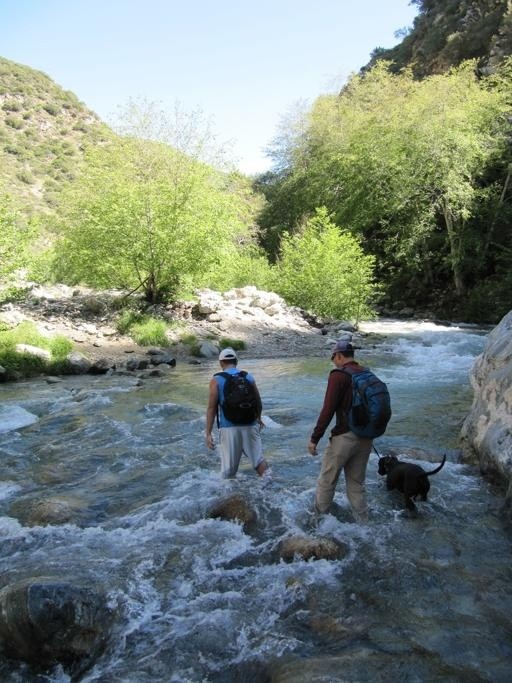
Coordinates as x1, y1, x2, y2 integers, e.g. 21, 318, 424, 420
202, 346, 272, 478
306, 338, 377, 525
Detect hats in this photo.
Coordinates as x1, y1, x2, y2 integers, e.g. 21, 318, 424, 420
332, 341, 354, 353
219, 347, 237, 361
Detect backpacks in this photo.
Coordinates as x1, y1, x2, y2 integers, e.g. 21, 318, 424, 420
330, 366, 391, 438
213, 370, 261, 425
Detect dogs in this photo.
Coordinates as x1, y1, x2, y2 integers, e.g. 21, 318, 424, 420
378, 453, 446, 512
